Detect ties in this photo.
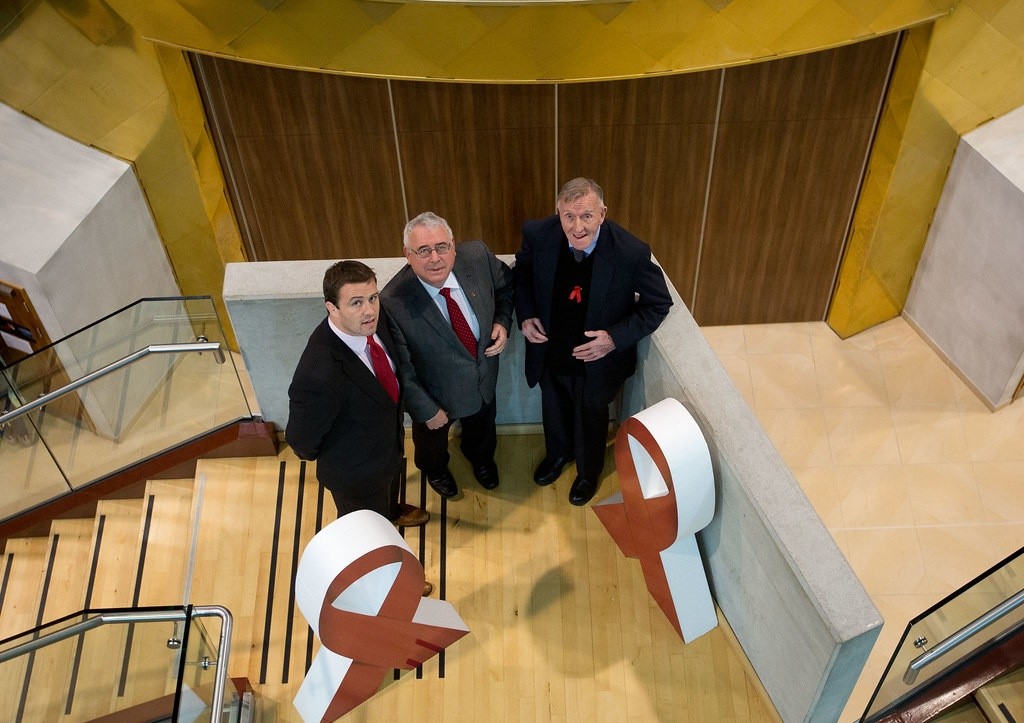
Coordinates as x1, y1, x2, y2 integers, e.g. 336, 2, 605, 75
438, 287, 479, 360
367, 335, 400, 405
573, 248, 583, 262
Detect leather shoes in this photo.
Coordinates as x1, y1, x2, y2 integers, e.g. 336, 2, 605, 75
470, 452, 500, 490
421, 463, 458, 498
533, 444, 577, 486
569, 475, 599, 506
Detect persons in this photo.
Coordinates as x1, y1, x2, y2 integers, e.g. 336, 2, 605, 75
513, 177, 674, 506
283, 260, 431, 595
377, 212, 512, 500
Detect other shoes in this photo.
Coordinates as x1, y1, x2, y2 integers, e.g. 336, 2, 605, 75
391, 503, 431, 527
421, 581, 433, 597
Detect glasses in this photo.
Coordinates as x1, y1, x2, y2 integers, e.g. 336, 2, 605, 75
411, 241, 453, 258
